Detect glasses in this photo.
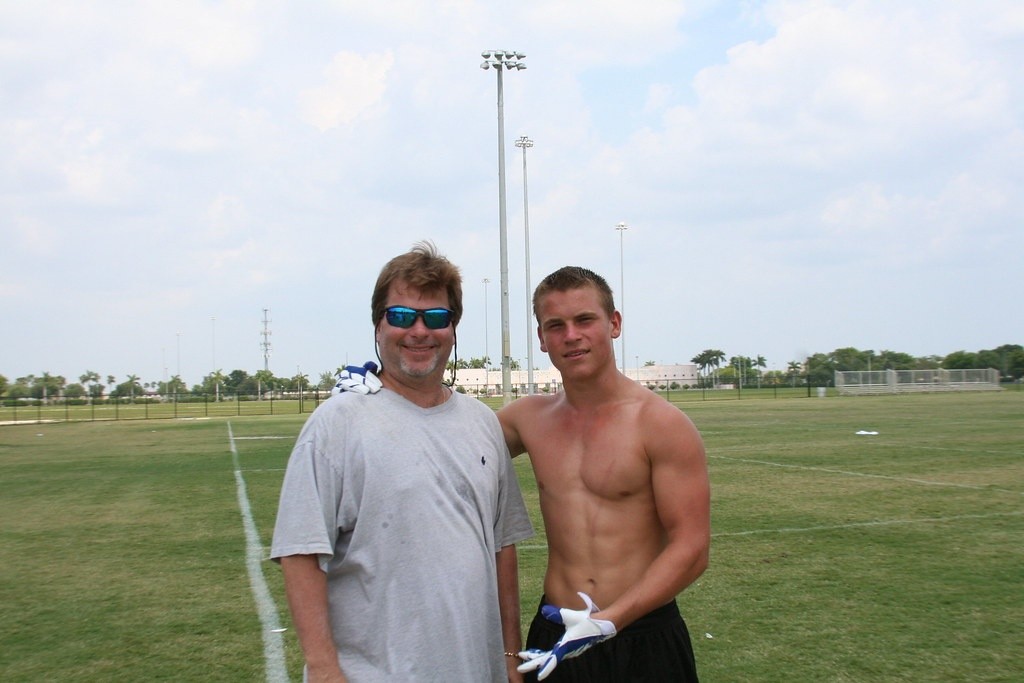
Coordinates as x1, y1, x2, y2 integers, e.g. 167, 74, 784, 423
382, 305, 455, 329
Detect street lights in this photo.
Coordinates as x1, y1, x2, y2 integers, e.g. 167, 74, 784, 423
480, 49, 526, 411
513, 134, 534, 395
615, 221, 628, 373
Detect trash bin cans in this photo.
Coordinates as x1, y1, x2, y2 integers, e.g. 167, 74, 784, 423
817, 387, 827, 397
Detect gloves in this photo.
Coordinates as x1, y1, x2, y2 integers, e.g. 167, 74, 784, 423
332, 361, 383, 395
516, 590, 617, 681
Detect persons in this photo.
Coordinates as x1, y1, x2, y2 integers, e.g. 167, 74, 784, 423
270, 241, 535, 683
332, 265, 712, 683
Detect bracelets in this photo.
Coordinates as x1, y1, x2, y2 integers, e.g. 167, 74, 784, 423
504, 652, 520, 658
